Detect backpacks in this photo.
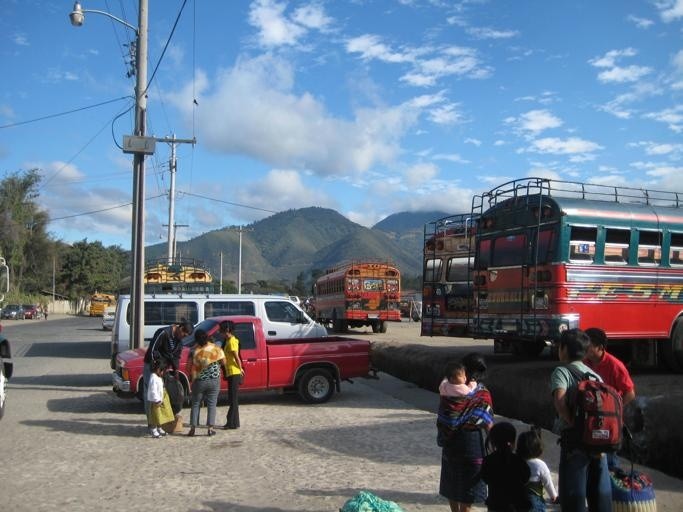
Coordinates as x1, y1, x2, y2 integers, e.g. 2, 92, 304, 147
566, 365, 625, 452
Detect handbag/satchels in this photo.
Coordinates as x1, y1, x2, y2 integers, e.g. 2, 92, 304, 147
612, 464, 656, 511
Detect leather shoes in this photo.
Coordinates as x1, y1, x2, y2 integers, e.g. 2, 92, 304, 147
209, 429, 215, 436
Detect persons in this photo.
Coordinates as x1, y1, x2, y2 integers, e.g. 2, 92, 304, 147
219, 320, 245, 430
187, 329, 227, 436
304, 298, 310, 311
31, 303, 48, 320
436, 328, 636, 512
142, 319, 194, 418
147, 358, 175, 438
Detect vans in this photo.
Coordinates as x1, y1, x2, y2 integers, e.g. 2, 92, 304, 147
109, 293, 327, 370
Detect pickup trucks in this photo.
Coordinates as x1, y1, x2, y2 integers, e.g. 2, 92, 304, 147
110, 315, 371, 404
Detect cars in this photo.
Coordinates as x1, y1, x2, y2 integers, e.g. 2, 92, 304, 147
0, 338, 15, 421
101, 308, 115, 329
0, 304, 43, 320
399, 292, 422, 321
284, 296, 300, 306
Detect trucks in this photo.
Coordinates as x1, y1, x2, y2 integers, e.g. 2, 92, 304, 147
89, 294, 116, 317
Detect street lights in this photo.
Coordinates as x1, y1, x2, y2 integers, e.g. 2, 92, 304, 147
67, 0, 135, 350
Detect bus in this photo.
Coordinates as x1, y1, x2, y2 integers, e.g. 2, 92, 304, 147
416, 212, 493, 341
464, 176, 681, 369
311, 256, 401, 333
116, 256, 215, 295
